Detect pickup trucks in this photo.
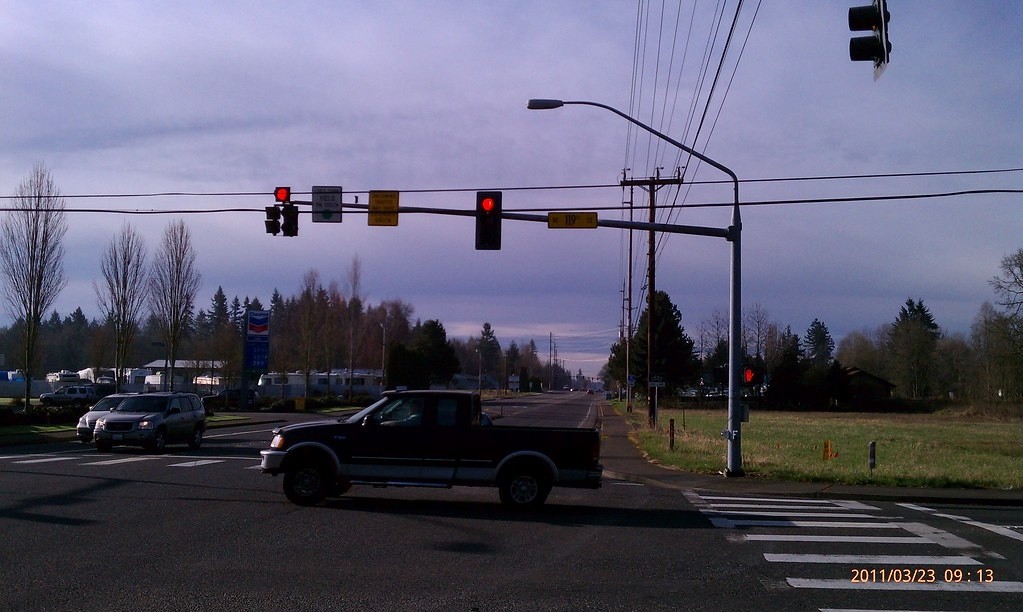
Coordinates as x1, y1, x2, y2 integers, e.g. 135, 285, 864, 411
260, 389, 606, 515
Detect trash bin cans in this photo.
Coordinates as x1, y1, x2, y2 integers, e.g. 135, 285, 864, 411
605, 392, 611, 400
295, 397, 305, 411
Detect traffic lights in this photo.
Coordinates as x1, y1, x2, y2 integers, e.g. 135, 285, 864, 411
476, 191, 502, 250
264, 206, 281, 236
275, 186, 291, 202
741, 368, 755, 384
846, 0, 891, 82
280, 206, 299, 236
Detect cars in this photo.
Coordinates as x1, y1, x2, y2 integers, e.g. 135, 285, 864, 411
587, 390, 593, 394
76, 391, 141, 444
563, 384, 570, 391
571, 387, 578, 392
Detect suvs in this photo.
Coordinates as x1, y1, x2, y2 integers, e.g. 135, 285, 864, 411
92, 389, 206, 454
40, 385, 88, 407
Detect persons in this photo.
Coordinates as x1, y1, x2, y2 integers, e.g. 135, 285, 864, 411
380, 400, 422, 426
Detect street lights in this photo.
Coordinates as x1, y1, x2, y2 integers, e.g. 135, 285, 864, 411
527, 97, 740, 476
504, 354, 509, 394
475, 349, 482, 393
378, 321, 386, 388
